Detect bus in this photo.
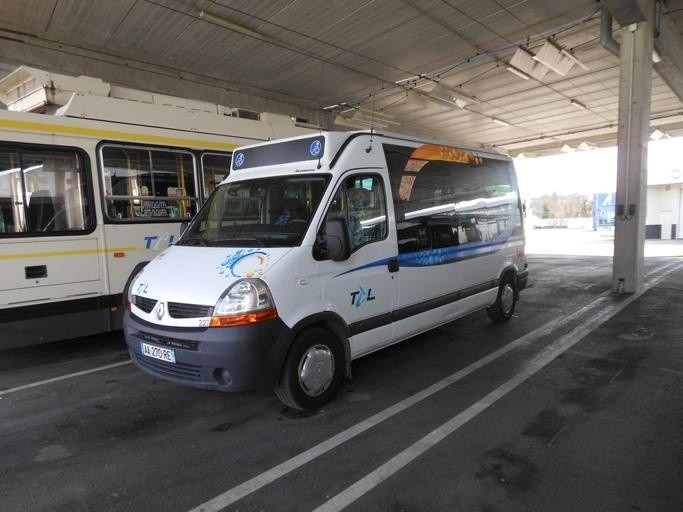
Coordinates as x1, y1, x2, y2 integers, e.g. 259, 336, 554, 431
0, 60, 323, 357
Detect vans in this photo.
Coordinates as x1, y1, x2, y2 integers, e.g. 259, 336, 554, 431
123, 129, 530, 416
